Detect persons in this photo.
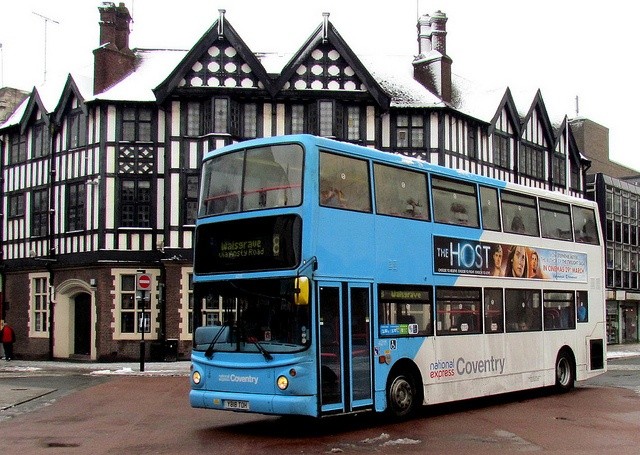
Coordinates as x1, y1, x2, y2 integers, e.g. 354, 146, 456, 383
531, 251, 544, 279
0, 319, 15, 360
491, 244, 505, 276
503, 244, 529, 278
518, 321, 529, 331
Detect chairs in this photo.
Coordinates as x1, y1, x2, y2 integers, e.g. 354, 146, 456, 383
485, 318, 493, 331
456, 315, 476, 332
548, 309, 563, 328
545, 314, 555, 328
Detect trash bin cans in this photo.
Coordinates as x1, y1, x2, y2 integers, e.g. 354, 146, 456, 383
167, 339, 178, 361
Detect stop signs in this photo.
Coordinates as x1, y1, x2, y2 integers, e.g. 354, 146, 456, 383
137, 274, 152, 291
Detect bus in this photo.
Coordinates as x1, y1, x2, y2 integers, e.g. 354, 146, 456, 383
189, 134, 609, 422
